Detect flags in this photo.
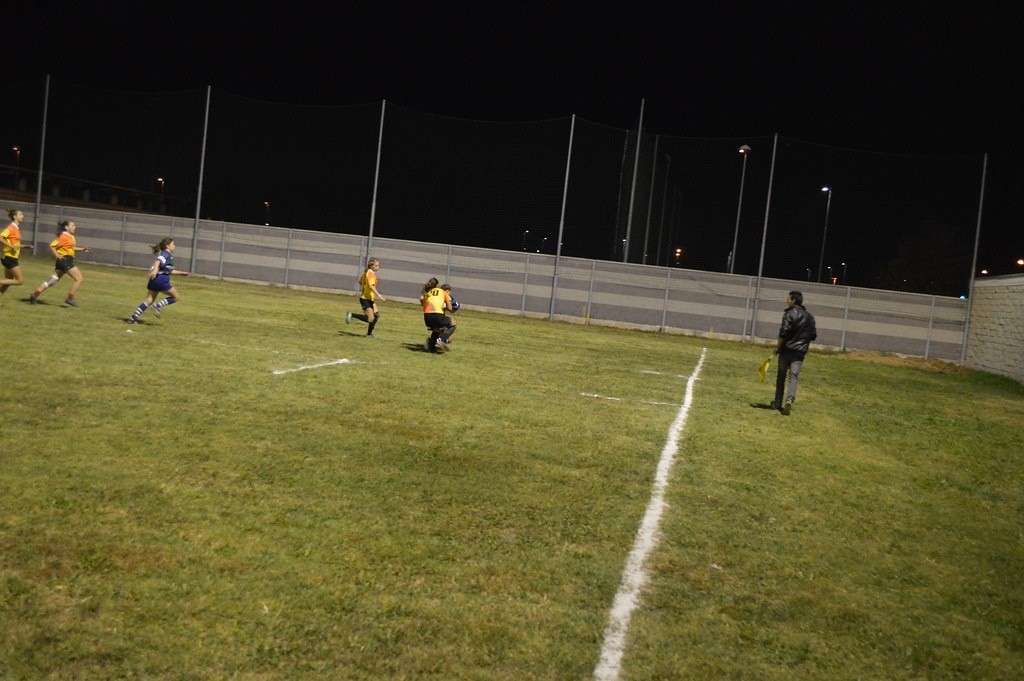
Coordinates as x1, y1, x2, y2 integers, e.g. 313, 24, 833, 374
758, 360, 769, 383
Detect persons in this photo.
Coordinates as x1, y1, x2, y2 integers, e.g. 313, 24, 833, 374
769, 290, 817, 414
126, 237, 191, 324
0, 209, 33, 294
345, 257, 387, 339
30, 219, 92, 307
419, 278, 461, 353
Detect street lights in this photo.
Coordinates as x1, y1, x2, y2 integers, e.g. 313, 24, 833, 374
263, 200, 270, 226
622, 239, 627, 255
13, 146, 22, 193
816, 186, 833, 283
675, 249, 681, 267
156, 177, 165, 210
839, 262, 850, 284
729, 144, 752, 274
540, 237, 548, 252
521, 230, 530, 252
827, 266, 834, 286
805, 268, 813, 281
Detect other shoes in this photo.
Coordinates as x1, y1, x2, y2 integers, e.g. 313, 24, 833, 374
66, 299, 78, 307
152, 303, 160, 318
128, 317, 139, 324
423, 337, 430, 349
345, 311, 352, 324
782, 401, 792, 416
365, 334, 377, 339
435, 338, 450, 353
770, 401, 780, 409
29, 293, 37, 305
